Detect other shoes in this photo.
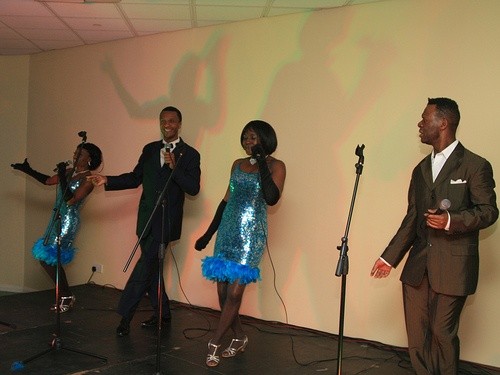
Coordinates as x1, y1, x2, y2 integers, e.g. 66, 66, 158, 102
50, 304, 59, 311
58, 295, 77, 313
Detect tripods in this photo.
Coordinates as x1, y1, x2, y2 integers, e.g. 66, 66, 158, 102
22, 130, 108, 365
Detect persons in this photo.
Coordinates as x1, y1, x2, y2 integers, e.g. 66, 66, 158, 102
370, 98, 499, 375
85, 106, 201, 336
11, 142, 102, 313
194, 120, 286, 366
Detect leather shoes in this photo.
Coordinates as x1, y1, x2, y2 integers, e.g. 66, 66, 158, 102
116, 324, 131, 336
140, 317, 168, 328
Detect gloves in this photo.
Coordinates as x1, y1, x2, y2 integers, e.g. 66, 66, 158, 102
195, 198, 228, 251
55, 161, 74, 202
10, 157, 51, 186
250, 143, 279, 206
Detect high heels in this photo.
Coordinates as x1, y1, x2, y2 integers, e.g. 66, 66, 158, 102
221, 334, 248, 358
206, 338, 220, 366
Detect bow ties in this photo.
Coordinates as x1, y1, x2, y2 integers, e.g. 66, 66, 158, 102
161, 143, 173, 149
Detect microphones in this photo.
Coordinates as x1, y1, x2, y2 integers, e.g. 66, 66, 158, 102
255, 148, 261, 160
424, 198, 451, 227
165, 143, 172, 153
53, 161, 70, 171
